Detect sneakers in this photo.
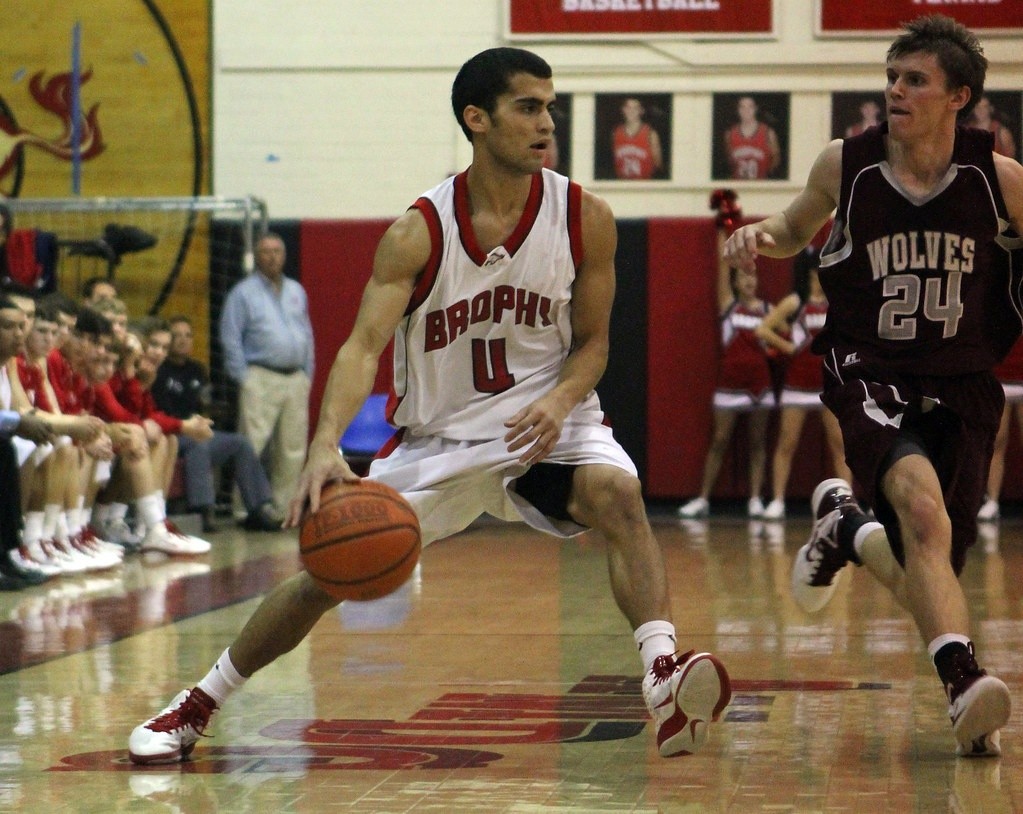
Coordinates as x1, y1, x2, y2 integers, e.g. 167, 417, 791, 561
790, 479, 865, 614
977, 499, 1001, 520
680, 495, 710, 522
1, 510, 218, 578
129, 773, 224, 811
762, 499, 786, 520
640, 650, 736, 756
128, 679, 220, 763
934, 645, 1012, 758
748, 494, 768, 519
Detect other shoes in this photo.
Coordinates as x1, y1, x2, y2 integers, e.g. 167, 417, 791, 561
201, 508, 220, 532
248, 507, 285, 531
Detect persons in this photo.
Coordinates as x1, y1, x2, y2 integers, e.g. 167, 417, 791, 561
0, 204, 282, 590
220, 234, 314, 518
613, 98, 662, 179
725, 96, 779, 180
130, 47, 732, 765
723, 17, 1023, 756
970, 95, 1014, 157
677, 225, 1023, 515
847, 101, 882, 138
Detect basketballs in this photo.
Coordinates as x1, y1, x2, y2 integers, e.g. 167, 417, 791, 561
297, 480, 420, 601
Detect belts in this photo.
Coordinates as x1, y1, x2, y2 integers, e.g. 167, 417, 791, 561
252, 361, 301, 375
